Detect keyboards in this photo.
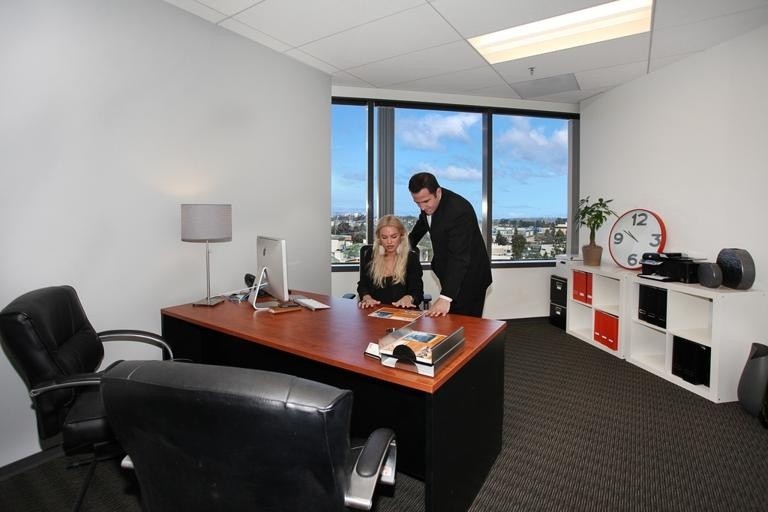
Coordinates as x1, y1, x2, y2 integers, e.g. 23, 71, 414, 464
295, 298, 331, 311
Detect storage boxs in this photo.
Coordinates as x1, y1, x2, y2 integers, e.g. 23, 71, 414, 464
549, 254, 585, 330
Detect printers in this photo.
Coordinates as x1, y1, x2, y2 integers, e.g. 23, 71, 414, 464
637, 252, 707, 284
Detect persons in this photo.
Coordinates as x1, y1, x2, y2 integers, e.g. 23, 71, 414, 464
355, 213, 424, 311
406, 171, 493, 321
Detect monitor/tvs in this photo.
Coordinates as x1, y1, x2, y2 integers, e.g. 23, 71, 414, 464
247, 235, 289, 310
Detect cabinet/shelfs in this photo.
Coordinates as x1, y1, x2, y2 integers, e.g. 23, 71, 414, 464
564, 265, 767, 406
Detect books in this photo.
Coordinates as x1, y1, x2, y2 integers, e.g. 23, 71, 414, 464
268, 305, 301, 316
367, 306, 424, 323
378, 338, 471, 377
379, 316, 465, 357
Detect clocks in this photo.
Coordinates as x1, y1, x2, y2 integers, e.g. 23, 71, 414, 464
608, 208, 668, 271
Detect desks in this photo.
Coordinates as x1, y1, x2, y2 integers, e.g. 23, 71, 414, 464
160, 287, 508, 511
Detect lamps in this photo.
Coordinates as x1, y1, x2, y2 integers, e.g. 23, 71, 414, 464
180, 202, 234, 307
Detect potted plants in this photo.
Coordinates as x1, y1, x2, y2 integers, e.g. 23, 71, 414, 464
572, 194, 621, 267
